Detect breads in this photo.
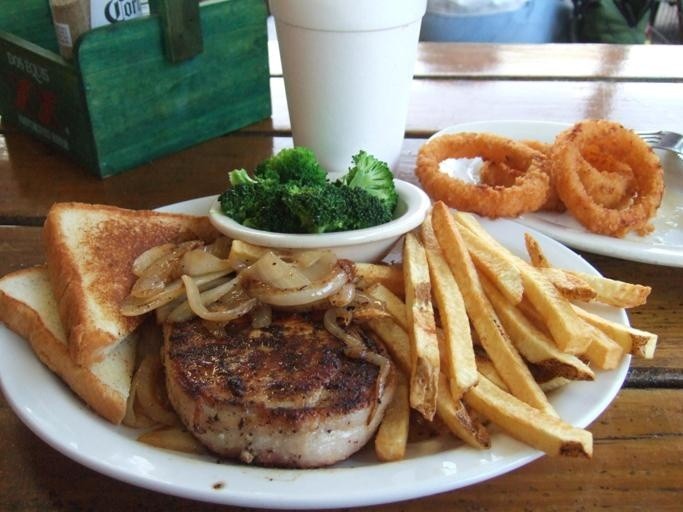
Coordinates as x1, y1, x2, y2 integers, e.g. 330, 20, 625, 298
44, 200, 228, 371
0, 261, 144, 422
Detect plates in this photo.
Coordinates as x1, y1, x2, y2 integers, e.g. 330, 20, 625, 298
211, 174, 429, 270
0, 185, 633, 510
423, 116, 682, 269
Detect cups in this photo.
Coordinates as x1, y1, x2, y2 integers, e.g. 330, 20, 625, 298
269, 1, 427, 183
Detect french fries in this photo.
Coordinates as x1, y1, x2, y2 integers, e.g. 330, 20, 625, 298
353, 201, 658, 461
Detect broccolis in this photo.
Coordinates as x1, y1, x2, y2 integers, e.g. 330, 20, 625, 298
219, 149, 400, 235
